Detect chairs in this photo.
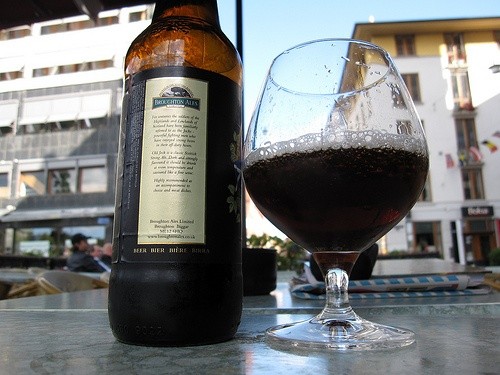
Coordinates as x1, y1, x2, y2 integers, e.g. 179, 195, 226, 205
0, 267, 110, 300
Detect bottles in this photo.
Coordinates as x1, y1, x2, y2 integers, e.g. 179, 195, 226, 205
108, 0, 243, 347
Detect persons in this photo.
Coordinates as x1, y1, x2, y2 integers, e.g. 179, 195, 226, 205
64, 232, 113, 273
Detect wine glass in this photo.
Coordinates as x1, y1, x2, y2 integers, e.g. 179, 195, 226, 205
244, 38, 429, 352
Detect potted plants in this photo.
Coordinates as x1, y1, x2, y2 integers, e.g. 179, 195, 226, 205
242, 234, 305, 296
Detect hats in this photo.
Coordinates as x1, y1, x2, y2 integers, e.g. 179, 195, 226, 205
71, 233, 92, 244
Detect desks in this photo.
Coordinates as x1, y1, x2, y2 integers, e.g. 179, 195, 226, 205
0, 258, 500, 375
